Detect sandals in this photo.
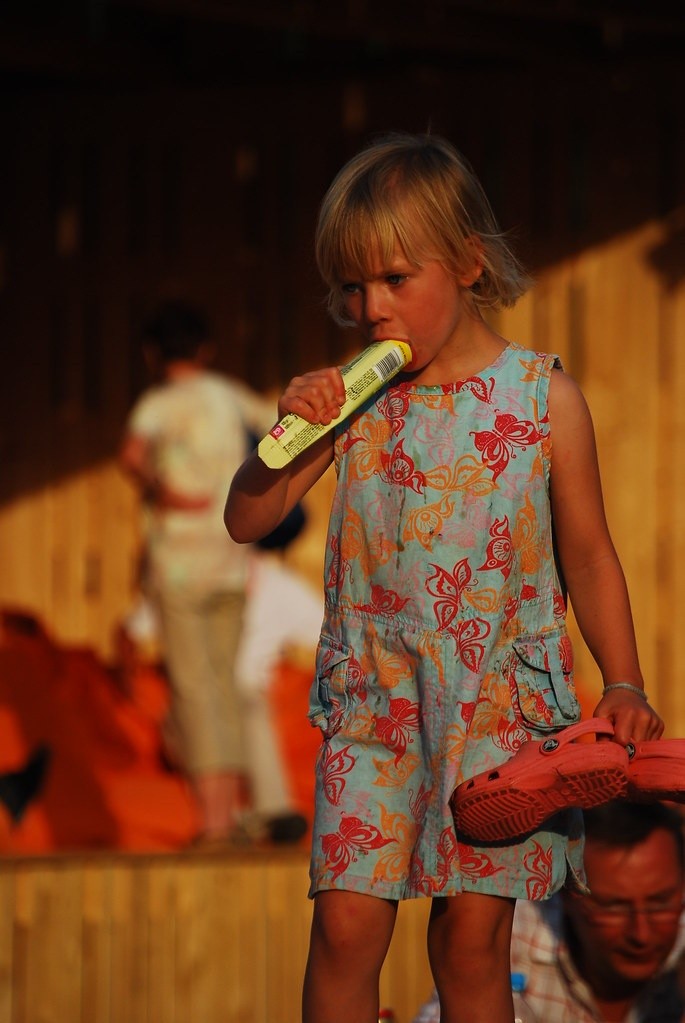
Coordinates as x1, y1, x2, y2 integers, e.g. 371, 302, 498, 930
449, 717, 632, 842
619, 737, 685, 792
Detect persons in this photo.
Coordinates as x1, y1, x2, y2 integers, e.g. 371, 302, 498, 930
411, 796, 685, 1023
115, 304, 327, 859
224, 129, 668, 1023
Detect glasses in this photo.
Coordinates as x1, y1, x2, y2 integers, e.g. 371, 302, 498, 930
568, 899, 681, 928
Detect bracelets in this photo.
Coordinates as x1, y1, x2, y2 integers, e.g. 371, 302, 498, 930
601, 683, 648, 699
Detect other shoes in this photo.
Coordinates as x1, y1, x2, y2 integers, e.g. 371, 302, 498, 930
267, 810, 307, 848
0, 742, 53, 822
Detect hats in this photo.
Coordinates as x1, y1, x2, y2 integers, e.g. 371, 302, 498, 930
253, 500, 305, 552
142, 296, 205, 357
